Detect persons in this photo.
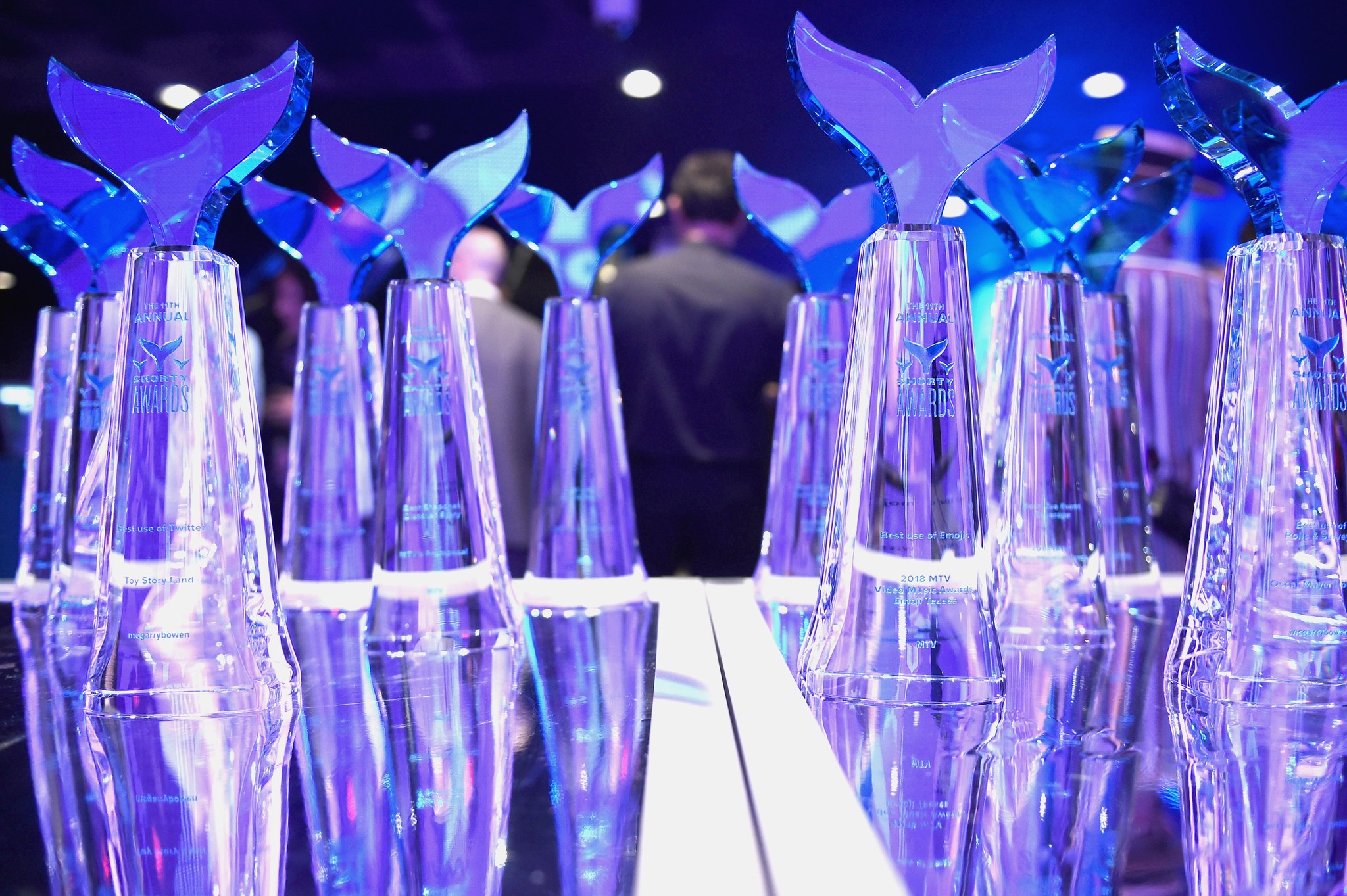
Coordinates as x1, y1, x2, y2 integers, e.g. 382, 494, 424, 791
264, 219, 547, 585
606, 149, 801, 578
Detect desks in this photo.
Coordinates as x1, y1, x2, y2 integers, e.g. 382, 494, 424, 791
634, 576, 918, 896
